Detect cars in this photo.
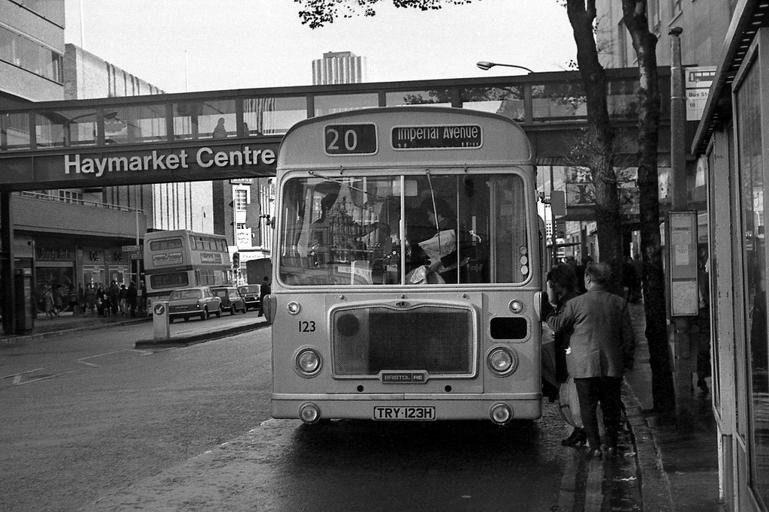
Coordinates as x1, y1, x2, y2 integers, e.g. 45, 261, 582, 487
166, 283, 263, 324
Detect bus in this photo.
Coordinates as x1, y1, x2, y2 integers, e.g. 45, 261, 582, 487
140, 229, 236, 317
245, 103, 567, 428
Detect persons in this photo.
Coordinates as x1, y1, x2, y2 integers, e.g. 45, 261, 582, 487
212, 118, 227, 139
256, 275, 271, 315
423, 197, 467, 285
540, 246, 711, 459
304, 193, 392, 267
39, 279, 146, 319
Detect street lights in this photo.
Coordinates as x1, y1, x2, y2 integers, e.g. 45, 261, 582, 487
477, 61, 558, 267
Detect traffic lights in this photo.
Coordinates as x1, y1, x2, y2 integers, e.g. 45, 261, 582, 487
233, 253, 240, 267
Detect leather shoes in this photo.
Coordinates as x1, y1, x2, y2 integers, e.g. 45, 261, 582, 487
562, 426, 617, 460
